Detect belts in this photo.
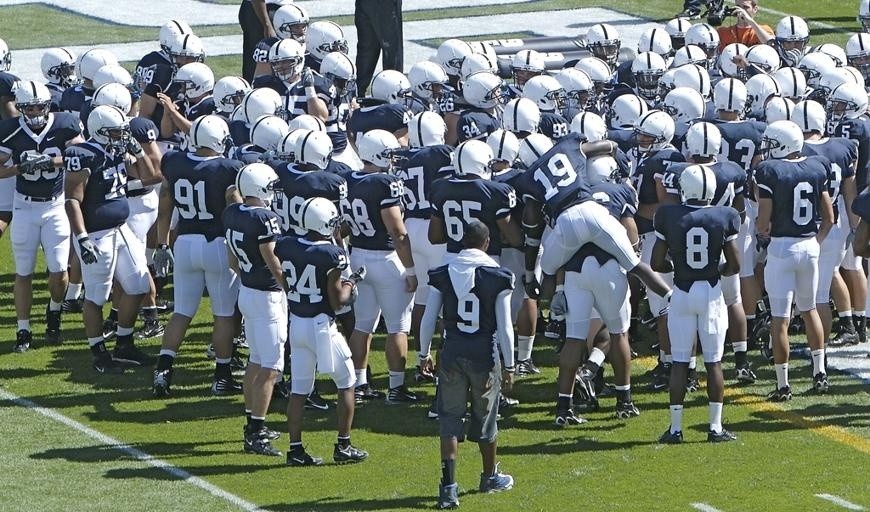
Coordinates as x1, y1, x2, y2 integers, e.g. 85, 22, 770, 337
23, 197, 57, 201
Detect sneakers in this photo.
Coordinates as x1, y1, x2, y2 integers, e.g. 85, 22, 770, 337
499, 392, 518, 409
93, 292, 174, 375
827, 327, 867, 345
545, 315, 561, 339
479, 475, 514, 493
738, 364, 756, 382
208, 344, 246, 359
302, 385, 330, 410
427, 401, 466, 423
465, 406, 501, 421
244, 434, 283, 456
615, 400, 640, 420
13, 329, 33, 352
233, 322, 249, 348
574, 361, 615, 412
211, 377, 243, 396
437, 482, 459, 509
354, 384, 387, 405
645, 360, 700, 393
270, 376, 293, 398
708, 428, 736, 442
333, 443, 367, 464
151, 369, 172, 398
43, 301, 64, 347
812, 373, 828, 393
658, 427, 683, 444
414, 364, 435, 383
757, 327, 775, 365
62, 297, 84, 313
766, 383, 792, 402
230, 354, 248, 370
286, 447, 323, 466
553, 410, 588, 427
243, 427, 281, 441
386, 382, 424, 403
516, 358, 540, 376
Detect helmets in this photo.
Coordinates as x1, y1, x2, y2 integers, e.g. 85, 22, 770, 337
160, 19, 193, 49
17, 47, 132, 154
407, 111, 448, 149
776, 16, 809, 43
610, 95, 648, 131
632, 17, 712, 120
761, 121, 804, 159
269, 1, 354, 96
174, 63, 333, 206
677, 164, 716, 201
519, 134, 554, 169
297, 196, 339, 239
438, 37, 612, 112
503, 98, 541, 134
858, 2, 870, 31
586, 152, 619, 185
452, 140, 494, 180
632, 110, 675, 153
568, 110, 608, 142
587, 23, 621, 66
712, 31, 870, 133
408, 61, 449, 106
369, 70, 412, 105
687, 122, 722, 158
0, 38, 13, 72
358, 129, 401, 168
169, 33, 205, 68
486, 129, 520, 168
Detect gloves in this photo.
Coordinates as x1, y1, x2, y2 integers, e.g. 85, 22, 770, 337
348, 265, 367, 284
550, 290, 567, 317
17, 152, 53, 174
844, 231, 863, 257
126, 137, 144, 157
340, 273, 358, 306
755, 230, 771, 251
77, 235, 102, 265
521, 273, 543, 298
152, 242, 174, 277
608, 147, 631, 173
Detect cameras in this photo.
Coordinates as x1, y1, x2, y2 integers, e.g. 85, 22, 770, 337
707, 0, 733, 26
